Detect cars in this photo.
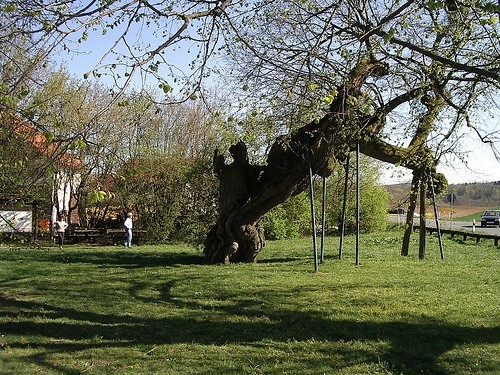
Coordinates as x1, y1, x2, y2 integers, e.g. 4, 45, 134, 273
480, 209, 500, 227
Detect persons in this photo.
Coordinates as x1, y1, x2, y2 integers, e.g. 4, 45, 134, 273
487, 213, 490, 215
124, 212, 134, 248
53, 216, 68, 250
